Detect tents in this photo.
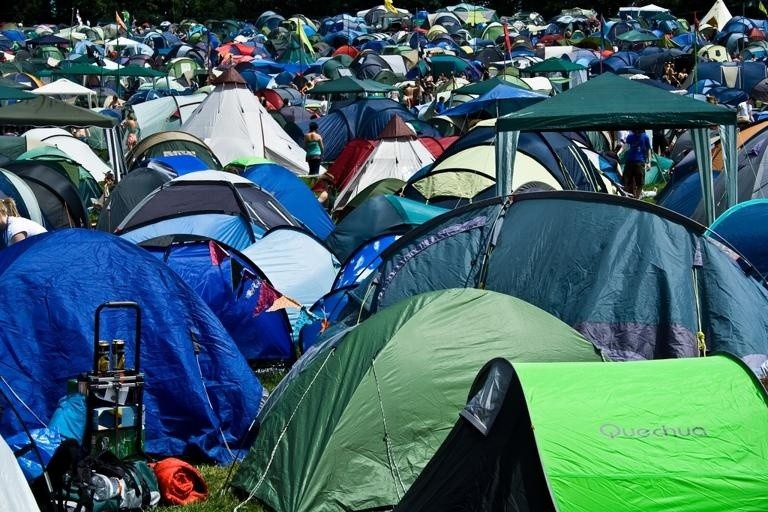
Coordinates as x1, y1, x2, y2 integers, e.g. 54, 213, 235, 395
0, 0, 768, 512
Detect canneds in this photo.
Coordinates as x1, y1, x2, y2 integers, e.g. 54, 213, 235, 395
112, 340, 125, 369
98, 340, 110, 372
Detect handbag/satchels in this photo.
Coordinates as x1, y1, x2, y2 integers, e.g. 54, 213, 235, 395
55, 450, 161, 511
